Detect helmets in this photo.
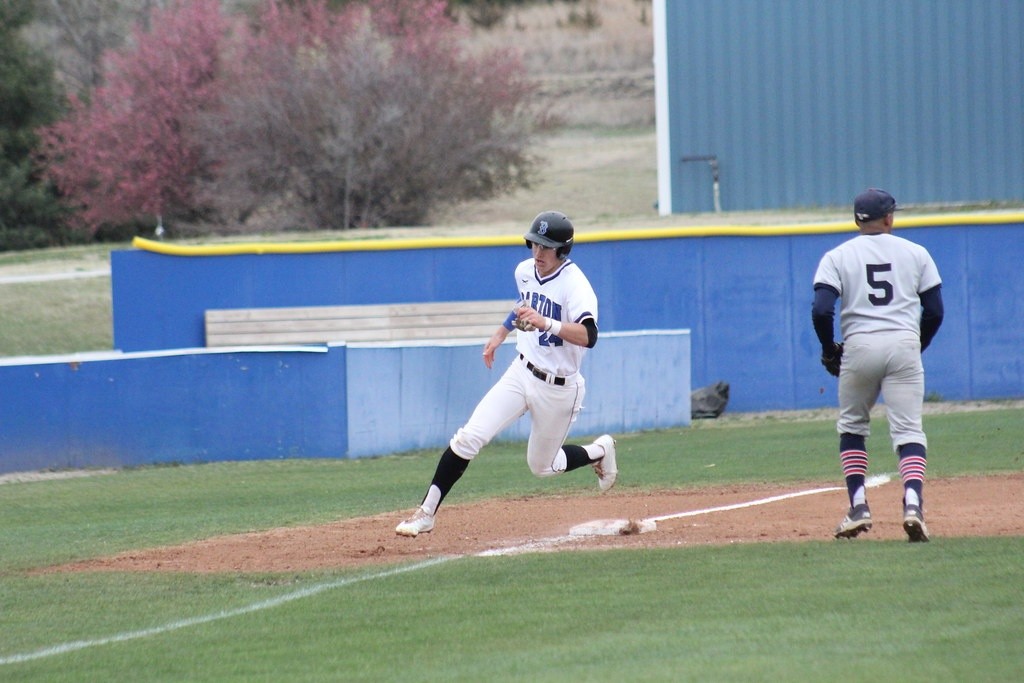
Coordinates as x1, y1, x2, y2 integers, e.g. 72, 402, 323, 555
524, 211, 574, 260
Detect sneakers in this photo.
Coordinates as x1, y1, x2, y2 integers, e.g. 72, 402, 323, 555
902, 504, 930, 543
833, 503, 872, 539
592, 434, 618, 491
396, 504, 434, 536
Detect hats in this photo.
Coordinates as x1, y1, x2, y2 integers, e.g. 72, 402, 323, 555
854, 188, 904, 223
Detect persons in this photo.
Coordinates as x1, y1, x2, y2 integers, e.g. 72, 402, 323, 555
812, 189, 944, 542
396, 211, 617, 536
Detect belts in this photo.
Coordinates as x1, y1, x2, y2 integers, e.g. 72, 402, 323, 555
520, 353, 565, 385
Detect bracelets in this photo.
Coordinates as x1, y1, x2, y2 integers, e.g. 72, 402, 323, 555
544, 317, 561, 336
